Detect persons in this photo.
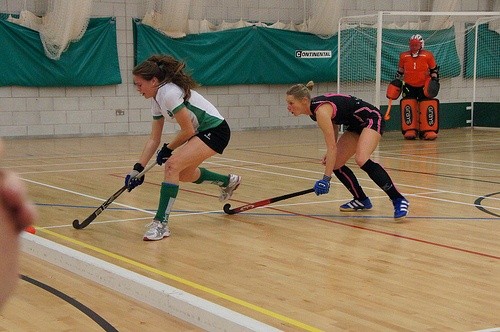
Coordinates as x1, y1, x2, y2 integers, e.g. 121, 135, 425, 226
285, 81, 411, 219
386, 34, 440, 140
126, 56, 242, 241
0, 167, 37, 311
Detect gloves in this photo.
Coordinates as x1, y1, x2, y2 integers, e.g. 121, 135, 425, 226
125, 163, 145, 193
313, 175, 331, 196
157, 144, 173, 166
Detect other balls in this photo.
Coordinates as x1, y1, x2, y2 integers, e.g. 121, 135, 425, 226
24, 226, 36, 234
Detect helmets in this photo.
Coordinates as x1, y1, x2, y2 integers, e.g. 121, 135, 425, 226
410, 34, 425, 54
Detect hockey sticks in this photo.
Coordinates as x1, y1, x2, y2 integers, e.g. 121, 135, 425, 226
223, 187, 316, 214
384, 97, 393, 120
72, 158, 156, 229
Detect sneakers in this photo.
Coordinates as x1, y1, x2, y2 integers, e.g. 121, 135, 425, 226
339, 197, 373, 211
392, 196, 410, 221
144, 221, 169, 241
218, 175, 241, 202
